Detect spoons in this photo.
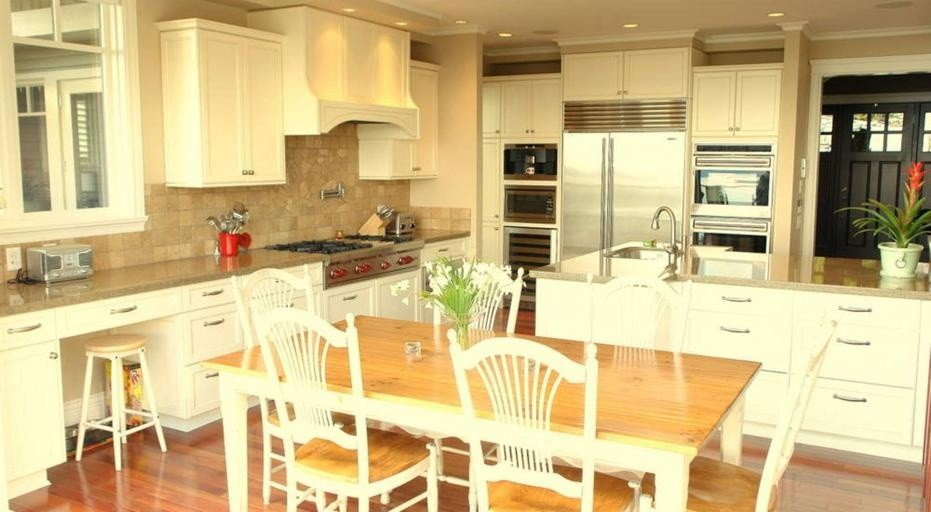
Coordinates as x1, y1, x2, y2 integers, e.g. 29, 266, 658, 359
206, 203, 253, 235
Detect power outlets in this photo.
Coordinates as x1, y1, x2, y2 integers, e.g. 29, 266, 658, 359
6, 247, 22, 270
799, 179, 804, 192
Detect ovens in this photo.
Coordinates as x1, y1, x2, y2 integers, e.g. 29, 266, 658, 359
688, 139, 777, 271
503, 141, 559, 228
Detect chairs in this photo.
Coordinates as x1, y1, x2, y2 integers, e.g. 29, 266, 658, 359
248, 302, 439, 512
231, 263, 355, 512
641, 322, 836, 511
585, 273, 693, 353
448, 329, 640, 512
380, 265, 524, 494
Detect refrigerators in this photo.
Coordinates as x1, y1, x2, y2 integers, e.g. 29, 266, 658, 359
558, 99, 687, 259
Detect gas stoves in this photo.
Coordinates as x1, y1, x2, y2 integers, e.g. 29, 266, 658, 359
266, 239, 426, 292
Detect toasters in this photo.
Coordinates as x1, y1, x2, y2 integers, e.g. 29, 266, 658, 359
24, 244, 95, 283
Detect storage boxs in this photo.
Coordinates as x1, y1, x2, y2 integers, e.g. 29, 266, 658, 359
104, 361, 144, 429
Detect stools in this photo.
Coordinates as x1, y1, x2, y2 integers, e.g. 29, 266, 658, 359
75, 335, 167, 471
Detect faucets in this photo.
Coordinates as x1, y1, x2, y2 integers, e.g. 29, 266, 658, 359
651, 206, 679, 276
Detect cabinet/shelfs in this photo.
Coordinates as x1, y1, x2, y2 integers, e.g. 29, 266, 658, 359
481, 226, 500, 263
564, 48, 688, 99
423, 237, 470, 324
791, 293, 920, 447
358, 60, 442, 179
242, 263, 324, 349
686, 285, 791, 428
481, 77, 501, 137
483, 139, 500, 225
154, 18, 287, 188
324, 268, 423, 323
0, 278, 244, 500
502, 75, 560, 138
692, 69, 781, 138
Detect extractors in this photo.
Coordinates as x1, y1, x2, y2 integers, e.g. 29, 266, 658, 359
248, 7, 422, 142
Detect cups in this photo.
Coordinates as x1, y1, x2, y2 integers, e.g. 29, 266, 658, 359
403, 341, 420, 362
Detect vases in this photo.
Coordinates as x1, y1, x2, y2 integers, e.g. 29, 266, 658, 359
878, 242, 924, 275
455, 322, 470, 350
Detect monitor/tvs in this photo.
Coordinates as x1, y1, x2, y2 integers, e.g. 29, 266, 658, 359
78, 171, 99, 208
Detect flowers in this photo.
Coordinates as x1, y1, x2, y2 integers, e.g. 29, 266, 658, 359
389, 249, 527, 323
834, 161, 931, 248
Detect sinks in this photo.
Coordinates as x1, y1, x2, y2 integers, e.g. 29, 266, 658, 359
604, 246, 672, 260
